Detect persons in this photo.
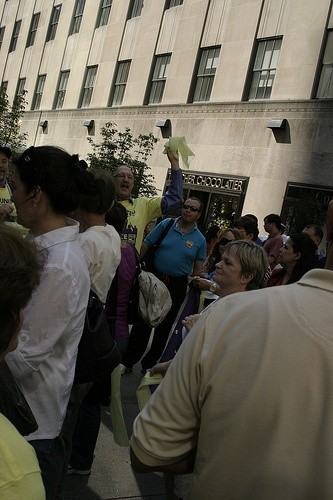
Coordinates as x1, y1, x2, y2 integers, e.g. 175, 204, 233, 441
47, 169, 118, 500
0, 146, 17, 221
68, 202, 136, 474
106, 146, 325, 376
0, 222, 49, 500
4, 145, 85, 500
129, 200, 333, 500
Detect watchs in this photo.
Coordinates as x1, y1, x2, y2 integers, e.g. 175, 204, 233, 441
210, 282, 217, 293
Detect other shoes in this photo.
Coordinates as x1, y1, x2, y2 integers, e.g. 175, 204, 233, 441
124, 367, 132, 373
65, 464, 92, 476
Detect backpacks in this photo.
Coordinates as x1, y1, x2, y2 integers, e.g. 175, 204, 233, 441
135, 266, 172, 325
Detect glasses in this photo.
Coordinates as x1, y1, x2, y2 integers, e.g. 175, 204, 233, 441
183, 204, 199, 212
219, 237, 233, 246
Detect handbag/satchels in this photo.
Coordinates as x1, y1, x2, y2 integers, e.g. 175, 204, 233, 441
74, 289, 119, 385
110, 364, 162, 447
127, 260, 148, 327
